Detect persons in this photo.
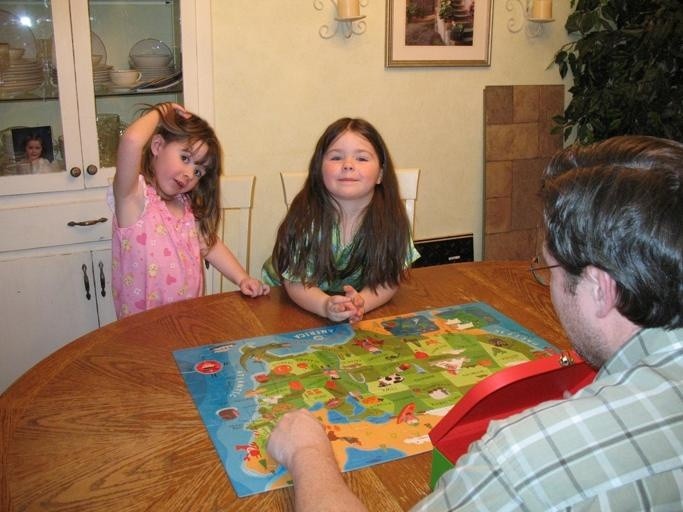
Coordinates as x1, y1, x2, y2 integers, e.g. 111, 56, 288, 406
19, 136, 52, 174
105, 101, 270, 321
265, 134, 683, 511
262, 118, 422, 325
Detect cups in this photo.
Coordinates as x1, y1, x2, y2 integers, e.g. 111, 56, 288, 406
94, 111, 129, 168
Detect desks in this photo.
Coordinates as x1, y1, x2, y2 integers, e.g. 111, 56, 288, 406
0, 258, 598, 511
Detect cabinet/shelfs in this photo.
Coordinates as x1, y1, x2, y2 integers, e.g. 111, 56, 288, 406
1, 0, 214, 193
1, 180, 210, 395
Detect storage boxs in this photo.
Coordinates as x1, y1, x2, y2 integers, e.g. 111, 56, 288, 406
426, 348, 598, 491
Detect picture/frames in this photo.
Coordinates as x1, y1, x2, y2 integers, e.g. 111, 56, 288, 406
384, 1, 495, 69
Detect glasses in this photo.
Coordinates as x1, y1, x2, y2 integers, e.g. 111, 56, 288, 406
530, 254, 560, 286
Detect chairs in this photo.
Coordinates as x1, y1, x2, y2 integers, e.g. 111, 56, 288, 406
278, 168, 421, 276
97, 175, 254, 300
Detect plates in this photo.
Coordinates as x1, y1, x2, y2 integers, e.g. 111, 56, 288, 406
0, 8, 182, 101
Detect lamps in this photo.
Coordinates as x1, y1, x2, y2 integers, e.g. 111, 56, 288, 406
506, 0, 558, 37
311, 0, 372, 40
